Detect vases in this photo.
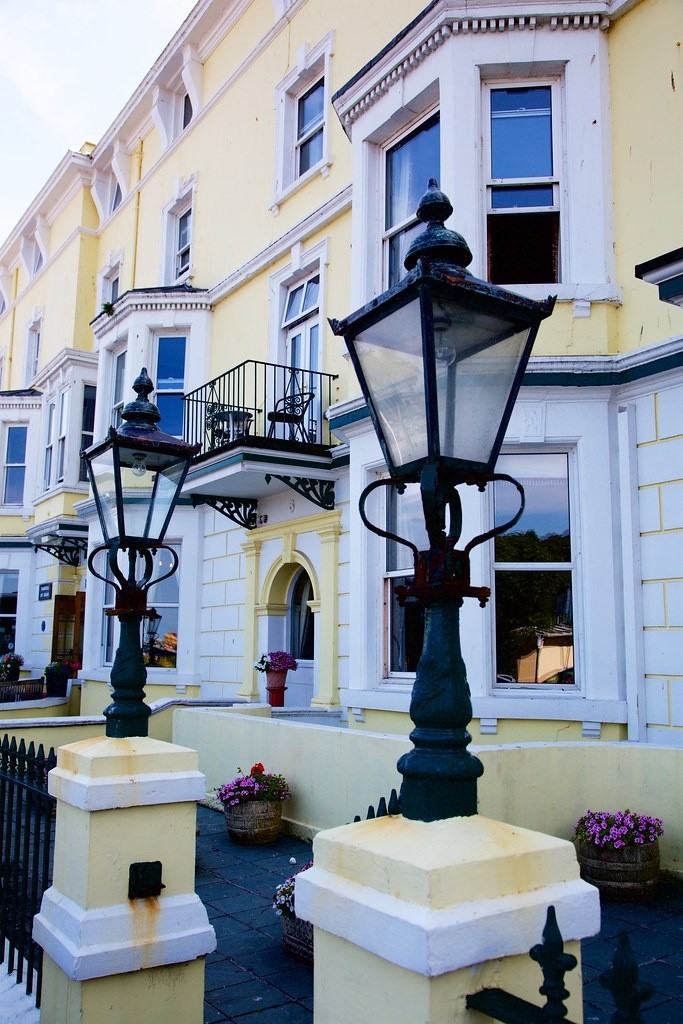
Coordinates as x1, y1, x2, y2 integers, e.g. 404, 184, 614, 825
47, 675, 68, 698
281, 912, 314, 968
265, 669, 287, 688
8, 668, 20, 681
574, 837, 658, 901
223, 801, 282, 845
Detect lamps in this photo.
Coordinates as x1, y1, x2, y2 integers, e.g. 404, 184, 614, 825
79, 367, 206, 736
326, 180, 558, 811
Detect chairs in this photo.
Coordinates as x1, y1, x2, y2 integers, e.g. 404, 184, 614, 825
267, 392, 315, 443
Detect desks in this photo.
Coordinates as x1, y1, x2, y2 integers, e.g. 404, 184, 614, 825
215, 410, 253, 448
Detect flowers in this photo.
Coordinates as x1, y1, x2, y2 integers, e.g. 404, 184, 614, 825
210, 762, 293, 808
45, 661, 74, 677
254, 651, 298, 671
574, 809, 664, 847
272, 861, 314, 914
0, 653, 24, 682
